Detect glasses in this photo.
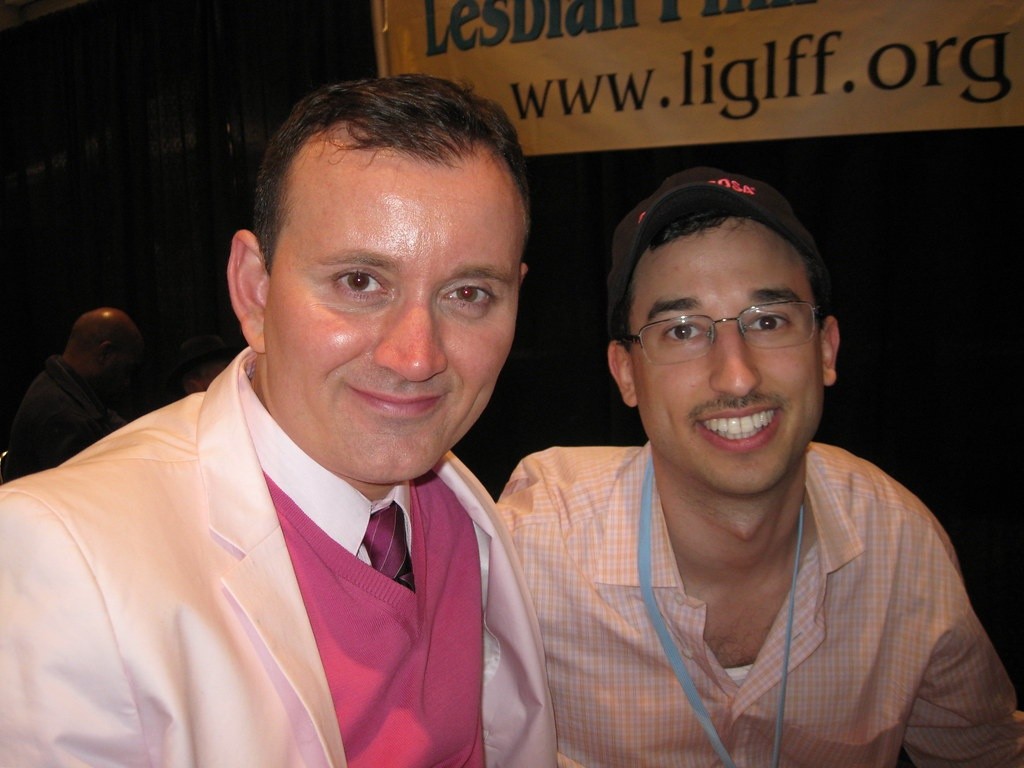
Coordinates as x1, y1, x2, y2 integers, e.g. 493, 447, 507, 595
629, 299, 816, 367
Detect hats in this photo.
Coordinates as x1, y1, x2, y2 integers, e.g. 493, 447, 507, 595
608, 165, 824, 336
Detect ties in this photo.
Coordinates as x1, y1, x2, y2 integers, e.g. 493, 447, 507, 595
366, 507, 415, 592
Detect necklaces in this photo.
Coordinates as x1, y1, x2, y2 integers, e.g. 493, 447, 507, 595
636, 455, 804, 768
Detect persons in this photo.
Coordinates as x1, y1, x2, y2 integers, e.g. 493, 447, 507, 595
5, 306, 143, 483
0, 73, 557, 768
168, 335, 239, 398
498, 171, 1024, 768
